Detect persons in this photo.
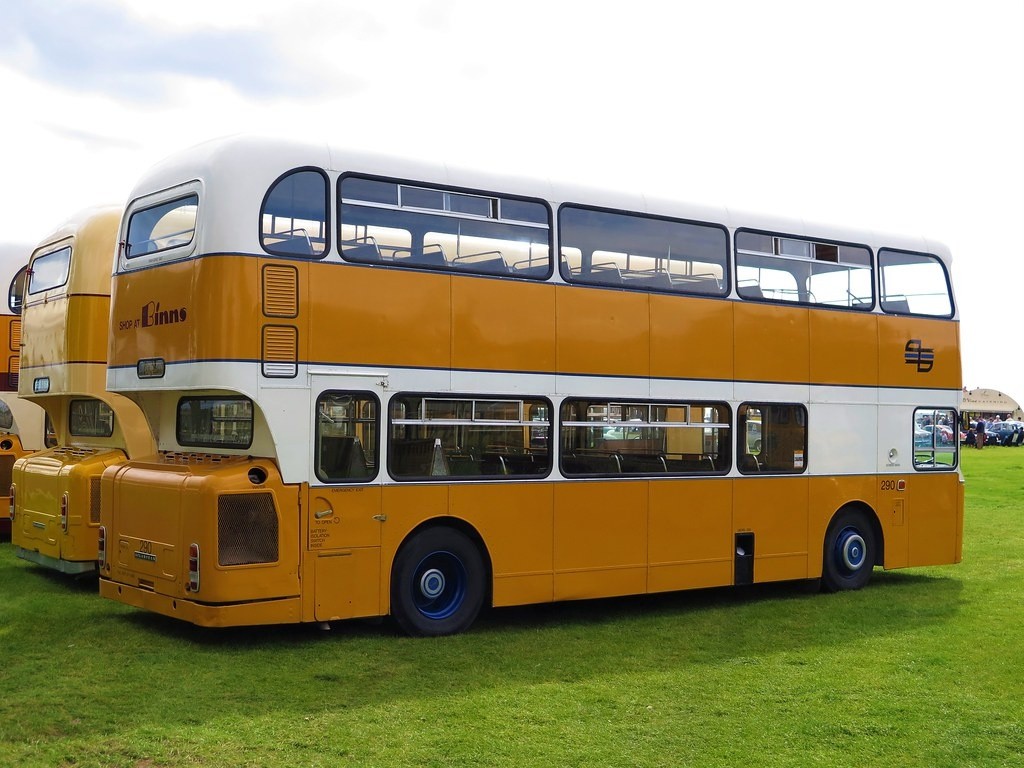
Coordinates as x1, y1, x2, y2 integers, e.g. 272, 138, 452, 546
921, 414, 1024, 450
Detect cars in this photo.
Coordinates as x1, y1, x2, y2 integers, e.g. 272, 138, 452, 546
988, 421, 1024, 439
922, 425, 967, 445
914, 420, 931, 447
600, 419, 644, 440
747, 420, 764, 454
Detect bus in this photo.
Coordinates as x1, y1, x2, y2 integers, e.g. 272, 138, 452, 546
0, 130, 966, 638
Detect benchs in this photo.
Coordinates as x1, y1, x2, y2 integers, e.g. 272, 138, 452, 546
454, 252, 508, 274
671, 273, 721, 292
738, 279, 766, 297
572, 262, 624, 285
322, 435, 481, 478
622, 268, 674, 289
481, 447, 760, 475
392, 244, 450, 266
852, 294, 914, 312
263, 228, 316, 257
512, 255, 574, 280
341, 237, 383, 262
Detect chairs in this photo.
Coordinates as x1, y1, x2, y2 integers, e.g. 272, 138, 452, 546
966, 438, 977, 447
